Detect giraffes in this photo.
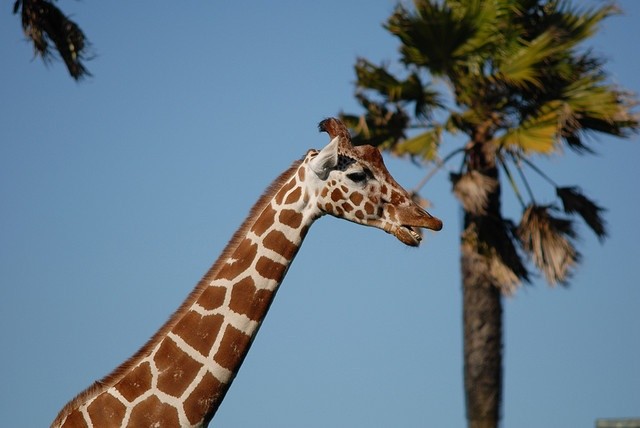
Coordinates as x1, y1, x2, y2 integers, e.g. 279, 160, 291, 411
49, 119, 444, 428
